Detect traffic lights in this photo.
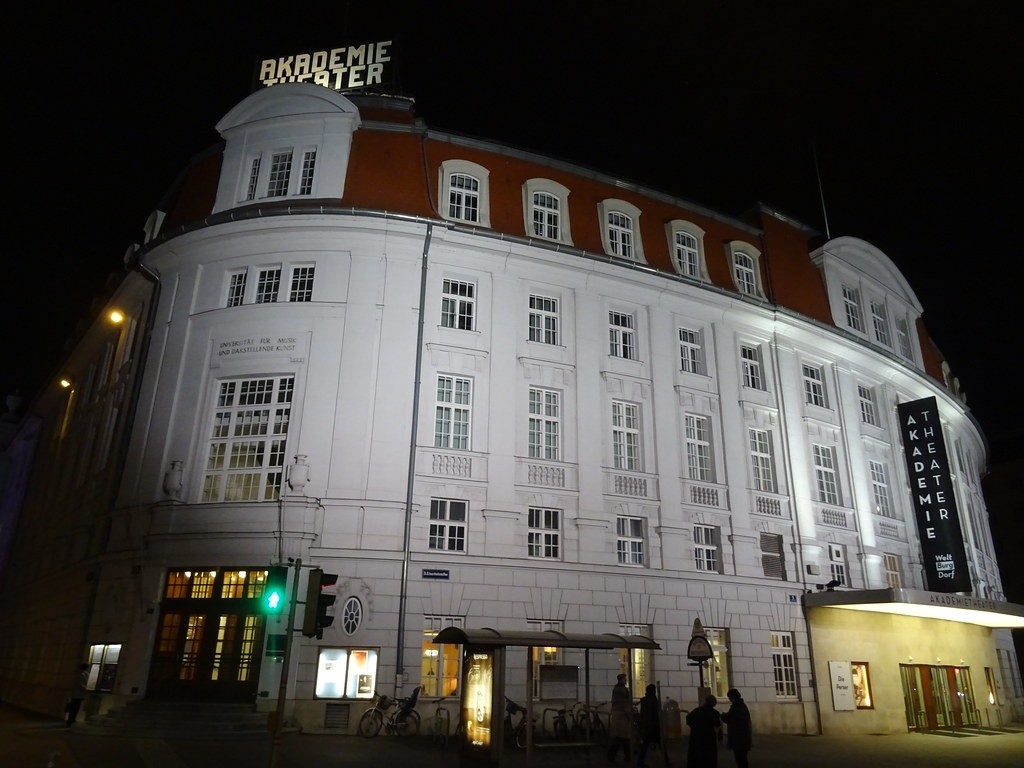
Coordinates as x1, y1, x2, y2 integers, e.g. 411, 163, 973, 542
303, 567, 338, 638
263, 566, 288, 613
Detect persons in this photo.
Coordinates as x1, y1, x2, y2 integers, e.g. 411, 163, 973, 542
606, 674, 633, 762
685, 695, 721, 768
636, 684, 672, 768
720, 689, 753, 768
67, 662, 90, 727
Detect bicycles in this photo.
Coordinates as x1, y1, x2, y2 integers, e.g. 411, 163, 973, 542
554, 700, 582, 742
576, 703, 608, 748
357, 691, 420, 739
503, 696, 536, 750
